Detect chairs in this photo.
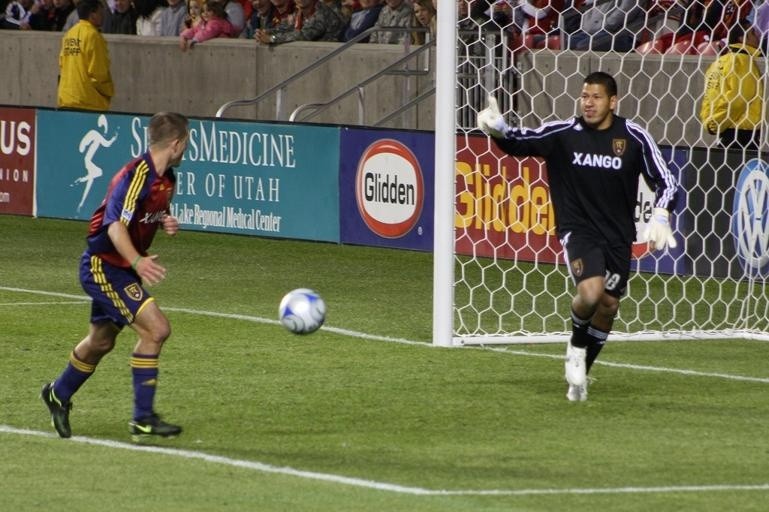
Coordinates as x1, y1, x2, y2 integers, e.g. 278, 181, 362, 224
507, 29, 726, 56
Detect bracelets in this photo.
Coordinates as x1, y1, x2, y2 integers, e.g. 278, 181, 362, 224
131, 255, 141, 270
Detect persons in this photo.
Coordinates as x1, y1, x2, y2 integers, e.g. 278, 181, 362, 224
459, 0, 769, 57
476, 72, 679, 402
460, 12, 518, 130
700, 19, 763, 150
57, 1, 114, 109
0, 1, 437, 45
41, 110, 182, 438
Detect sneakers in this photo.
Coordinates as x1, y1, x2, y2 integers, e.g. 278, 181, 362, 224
41, 384, 73, 438
565, 335, 588, 387
129, 414, 180, 436
566, 384, 588, 402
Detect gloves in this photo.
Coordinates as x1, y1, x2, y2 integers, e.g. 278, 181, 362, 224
643, 209, 677, 250
477, 95, 507, 138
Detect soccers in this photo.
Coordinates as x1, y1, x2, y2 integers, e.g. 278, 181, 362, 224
280, 288, 323, 334
